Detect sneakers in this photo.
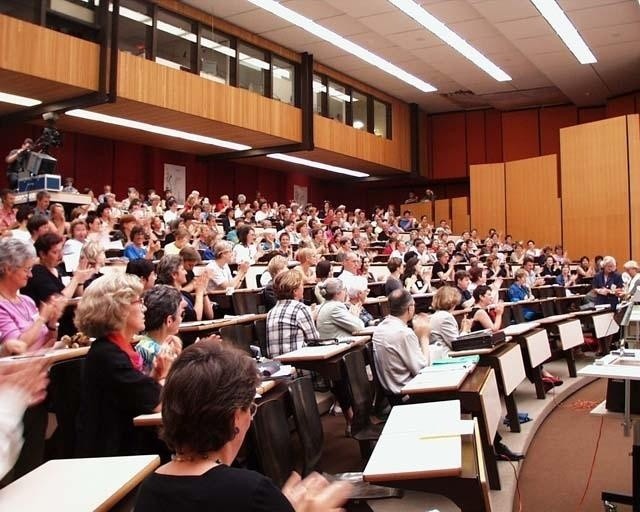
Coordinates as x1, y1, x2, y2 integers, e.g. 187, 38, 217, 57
332, 406, 344, 416
542, 374, 564, 386
542, 377, 554, 394
495, 442, 527, 462
344, 423, 354, 439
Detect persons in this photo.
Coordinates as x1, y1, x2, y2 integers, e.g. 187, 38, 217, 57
6, 138, 41, 186
64, 177, 79, 193
1, 185, 639, 512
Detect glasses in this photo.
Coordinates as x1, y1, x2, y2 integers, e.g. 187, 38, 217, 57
131, 297, 144, 309
